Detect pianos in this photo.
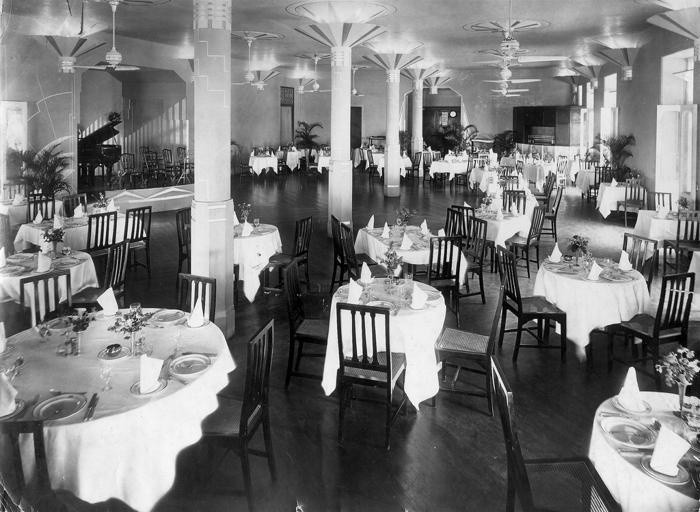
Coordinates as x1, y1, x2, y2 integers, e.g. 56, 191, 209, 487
78, 121, 121, 189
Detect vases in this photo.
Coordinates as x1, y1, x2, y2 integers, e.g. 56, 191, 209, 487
676, 383, 690, 414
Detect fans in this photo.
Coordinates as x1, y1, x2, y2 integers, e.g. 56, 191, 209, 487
474, 1, 569, 101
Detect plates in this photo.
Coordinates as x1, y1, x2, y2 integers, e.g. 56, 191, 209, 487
338, 278, 442, 314
0, 253, 88, 274
0, 308, 211, 422
599, 395, 690, 486
543, 256, 633, 282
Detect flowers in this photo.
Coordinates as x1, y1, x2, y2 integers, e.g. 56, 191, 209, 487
654, 342, 699, 391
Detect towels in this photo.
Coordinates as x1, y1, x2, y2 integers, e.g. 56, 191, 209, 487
137, 353, 165, 391
649, 424, 689, 477
618, 365, 645, 411
0, 371, 19, 418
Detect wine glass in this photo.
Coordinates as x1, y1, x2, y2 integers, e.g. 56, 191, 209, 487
82, 213, 88, 221
397, 219, 401, 226
63, 247, 71, 256
254, 218, 260, 228
98, 303, 181, 391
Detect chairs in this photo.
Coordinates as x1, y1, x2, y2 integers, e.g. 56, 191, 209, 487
605, 272, 696, 384
586, 387, 699, 511
486, 354, 619, 511
431, 274, 509, 415
0, 419, 69, 512
497, 243, 567, 367
0, 145, 194, 324
177, 272, 216, 323
182, 318, 274, 512
335, 303, 404, 449
275, 259, 330, 402
